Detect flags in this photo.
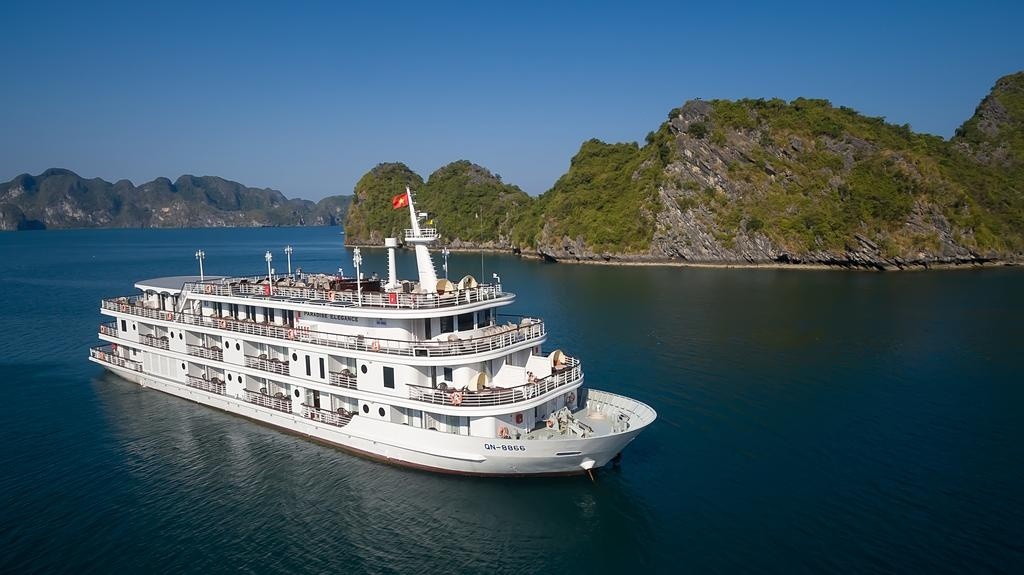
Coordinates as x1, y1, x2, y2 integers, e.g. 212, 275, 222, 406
391, 193, 409, 209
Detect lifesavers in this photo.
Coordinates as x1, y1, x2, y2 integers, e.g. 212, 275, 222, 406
167, 313, 172, 321
327, 291, 335, 301
221, 319, 227, 329
548, 420, 553, 428
205, 284, 212, 294
372, 339, 382, 352
450, 392, 462, 405
121, 304, 126, 312
101, 326, 104, 334
569, 392, 576, 402
99, 352, 105, 360
497, 426, 508, 438
288, 329, 294, 340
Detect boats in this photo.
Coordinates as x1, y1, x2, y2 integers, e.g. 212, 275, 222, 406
88, 186, 658, 475
